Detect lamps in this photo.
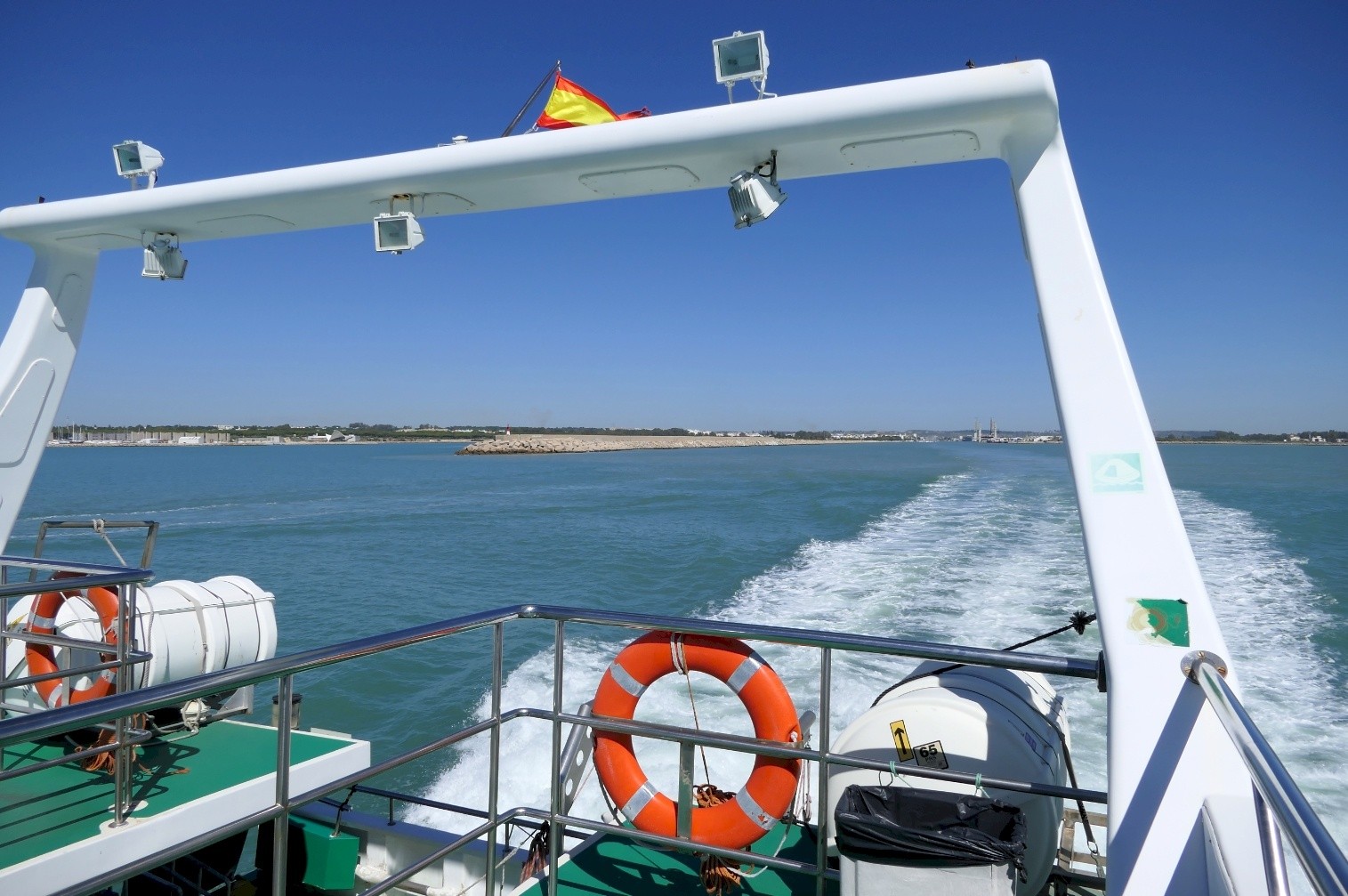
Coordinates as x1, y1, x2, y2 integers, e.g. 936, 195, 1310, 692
712, 30, 770, 103
110, 139, 165, 190
728, 150, 787, 228
141, 231, 189, 281
373, 194, 424, 256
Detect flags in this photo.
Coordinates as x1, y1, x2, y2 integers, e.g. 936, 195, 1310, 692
536, 70, 651, 129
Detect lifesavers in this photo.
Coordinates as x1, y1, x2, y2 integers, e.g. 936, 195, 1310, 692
590, 628, 804, 850
24, 571, 132, 711
